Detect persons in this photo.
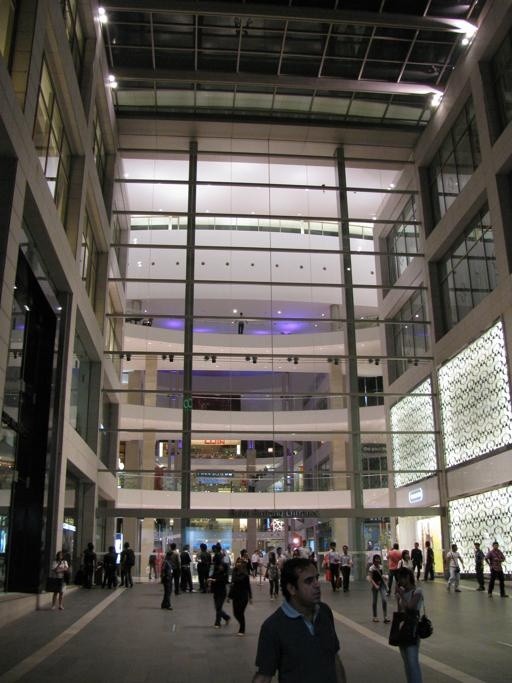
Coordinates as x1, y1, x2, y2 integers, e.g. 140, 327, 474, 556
446, 545, 466, 593
368, 554, 391, 623
388, 565, 425, 681
51, 551, 69, 612
81, 542, 135, 590
264, 540, 313, 598
474, 542, 486, 590
233, 310, 249, 334
148, 550, 158, 579
485, 541, 508, 598
245, 473, 256, 491
161, 543, 261, 636
250, 557, 347, 682
387, 541, 434, 596
325, 542, 352, 592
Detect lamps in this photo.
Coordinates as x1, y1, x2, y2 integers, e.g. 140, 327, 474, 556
117, 352, 420, 367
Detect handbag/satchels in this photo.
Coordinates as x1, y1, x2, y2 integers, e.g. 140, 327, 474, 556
45, 577, 62, 591
336, 576, 343, 589
419, 616, 434, 638
387, 610, 419, 647
